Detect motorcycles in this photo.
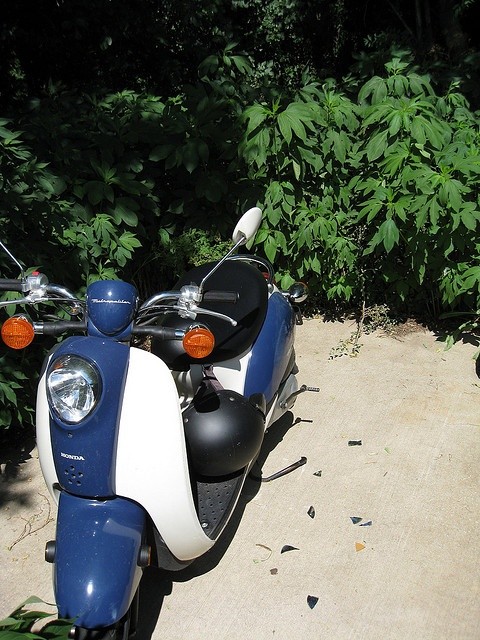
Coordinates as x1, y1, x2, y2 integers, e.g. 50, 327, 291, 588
0, 207, 321, 640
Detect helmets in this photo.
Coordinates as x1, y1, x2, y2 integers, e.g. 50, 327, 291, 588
183, 388, 265, 480
